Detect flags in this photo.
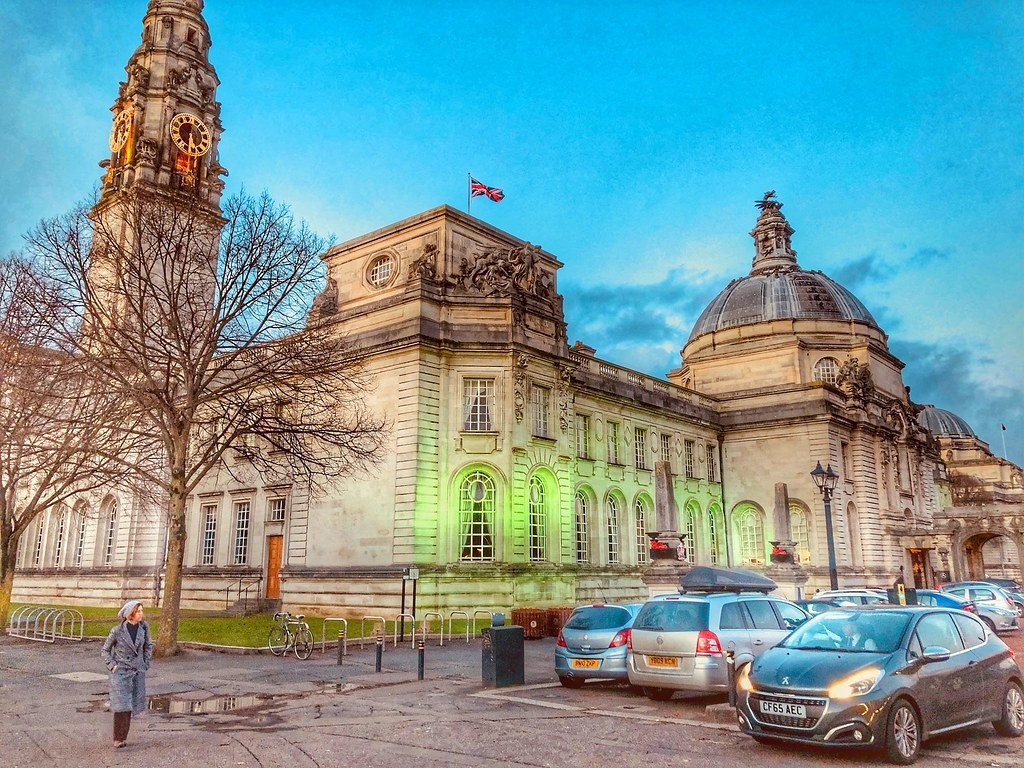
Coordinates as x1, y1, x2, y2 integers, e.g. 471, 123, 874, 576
470, 177, 505, 202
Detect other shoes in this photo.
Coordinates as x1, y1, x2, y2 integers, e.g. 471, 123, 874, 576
114, 742, 127, 748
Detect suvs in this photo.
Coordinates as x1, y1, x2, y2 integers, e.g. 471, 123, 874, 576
982, 579, 1022, 594
940, 581, 1024, 618
817, 595, 925, 607
814, 590, 877, 595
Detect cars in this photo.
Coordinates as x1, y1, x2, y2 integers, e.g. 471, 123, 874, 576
792, 599, 840, 618
945, 586, 1019, 633
737, 607, 1024, 764
873, 589, 887, 597
917, 587, 979, 618
555, 603, 643, 690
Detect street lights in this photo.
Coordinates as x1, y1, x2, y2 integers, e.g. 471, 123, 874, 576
810, 460, 841, 591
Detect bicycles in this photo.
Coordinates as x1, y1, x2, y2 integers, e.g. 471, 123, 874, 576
267, 611, 314, 661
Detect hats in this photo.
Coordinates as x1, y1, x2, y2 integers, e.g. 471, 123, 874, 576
119, 600, 141, 620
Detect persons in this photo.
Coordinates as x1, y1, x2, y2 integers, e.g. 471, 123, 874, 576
100, 599, 152, 749
839, 619, 880, 651
410, 241, 549, 292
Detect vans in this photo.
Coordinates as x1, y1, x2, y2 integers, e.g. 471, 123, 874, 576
625, 566, 815, 701
